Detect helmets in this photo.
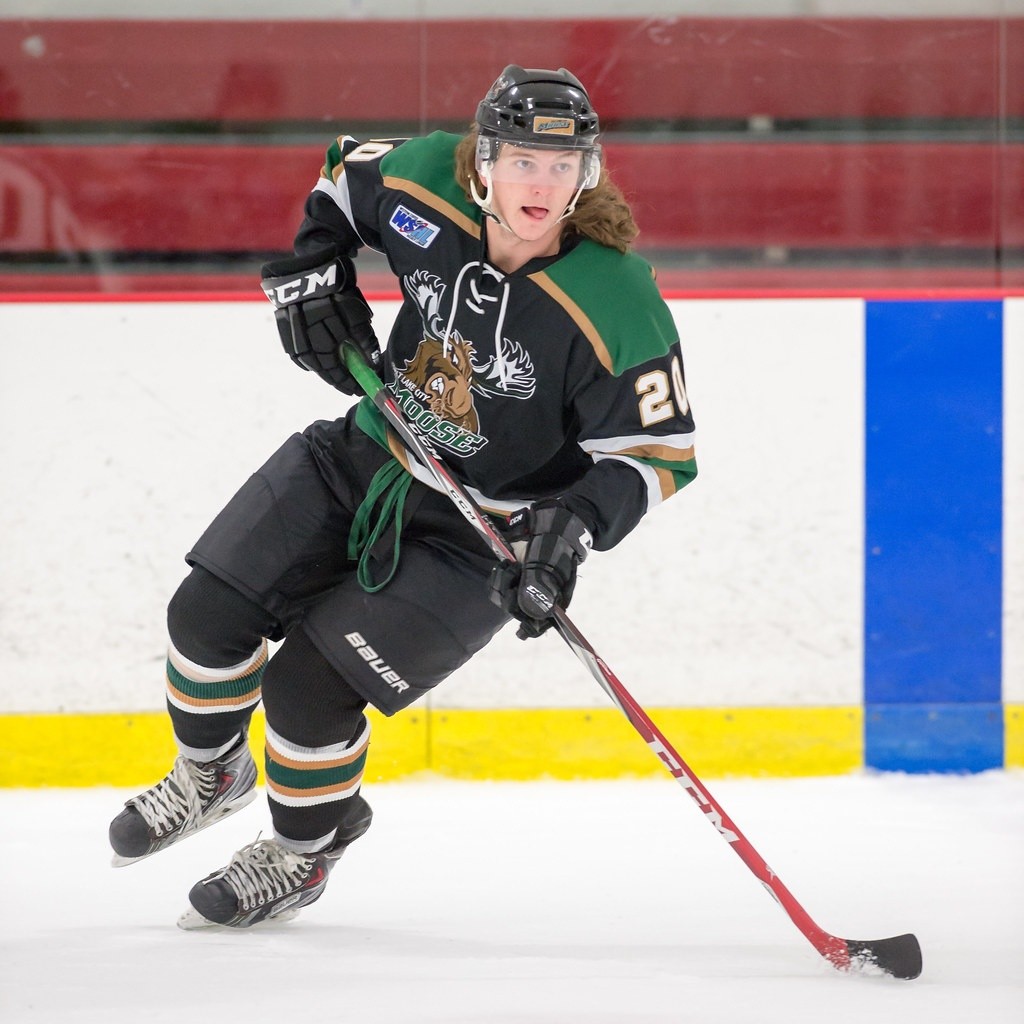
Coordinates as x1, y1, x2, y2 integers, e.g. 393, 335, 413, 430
475, 65, 602, 175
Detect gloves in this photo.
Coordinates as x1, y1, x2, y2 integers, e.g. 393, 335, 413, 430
260, 240, 385, 397
487, 496, 593, 641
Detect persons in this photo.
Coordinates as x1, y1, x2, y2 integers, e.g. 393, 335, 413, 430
108, 65, 700, 930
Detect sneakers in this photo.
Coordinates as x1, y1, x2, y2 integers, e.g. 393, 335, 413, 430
176, 796, 373, 933
108, 714, 259, 868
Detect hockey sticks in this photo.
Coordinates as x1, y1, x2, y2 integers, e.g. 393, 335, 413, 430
327, 333, 928, 987
